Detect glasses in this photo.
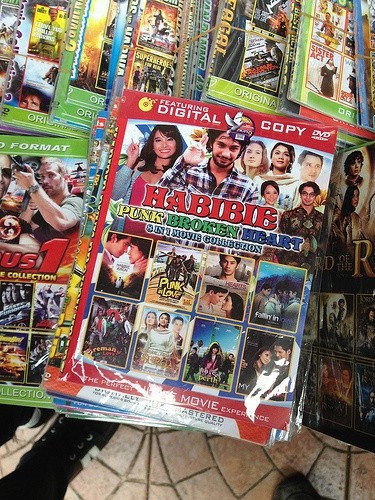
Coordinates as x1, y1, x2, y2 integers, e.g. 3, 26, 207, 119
0, 167, 12, 179
301, 191, 316, 198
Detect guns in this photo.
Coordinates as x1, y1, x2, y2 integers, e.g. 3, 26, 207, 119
10, 155, 41, 212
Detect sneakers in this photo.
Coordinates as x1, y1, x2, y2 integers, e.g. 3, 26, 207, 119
20, 408, 54, 428
40, 413, 121, 480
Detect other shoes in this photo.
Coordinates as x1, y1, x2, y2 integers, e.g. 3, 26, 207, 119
271, 471, 318, 500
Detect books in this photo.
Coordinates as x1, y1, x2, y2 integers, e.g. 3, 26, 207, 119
0, 2, 374, 451
